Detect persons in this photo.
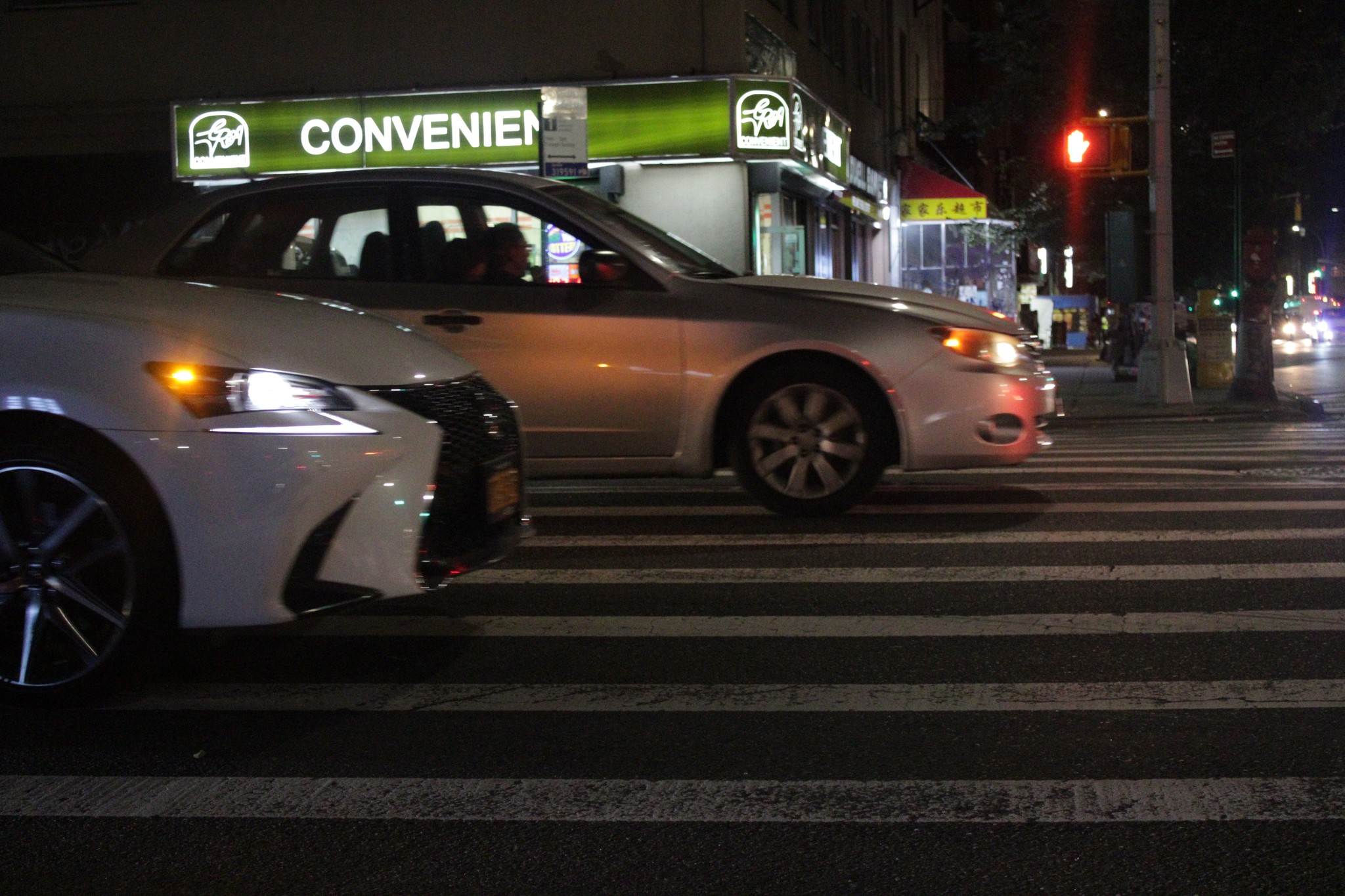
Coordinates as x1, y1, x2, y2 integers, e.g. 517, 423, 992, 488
471, 223, 547, 283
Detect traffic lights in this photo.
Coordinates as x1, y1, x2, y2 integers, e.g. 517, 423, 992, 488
1061, 124, 1115, 170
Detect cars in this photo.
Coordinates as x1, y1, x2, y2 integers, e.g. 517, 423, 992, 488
1272, 311, 1316, 341
77, 164, 1067, 517
0, 230, 536, 699
1315, 306, 1345, 344
